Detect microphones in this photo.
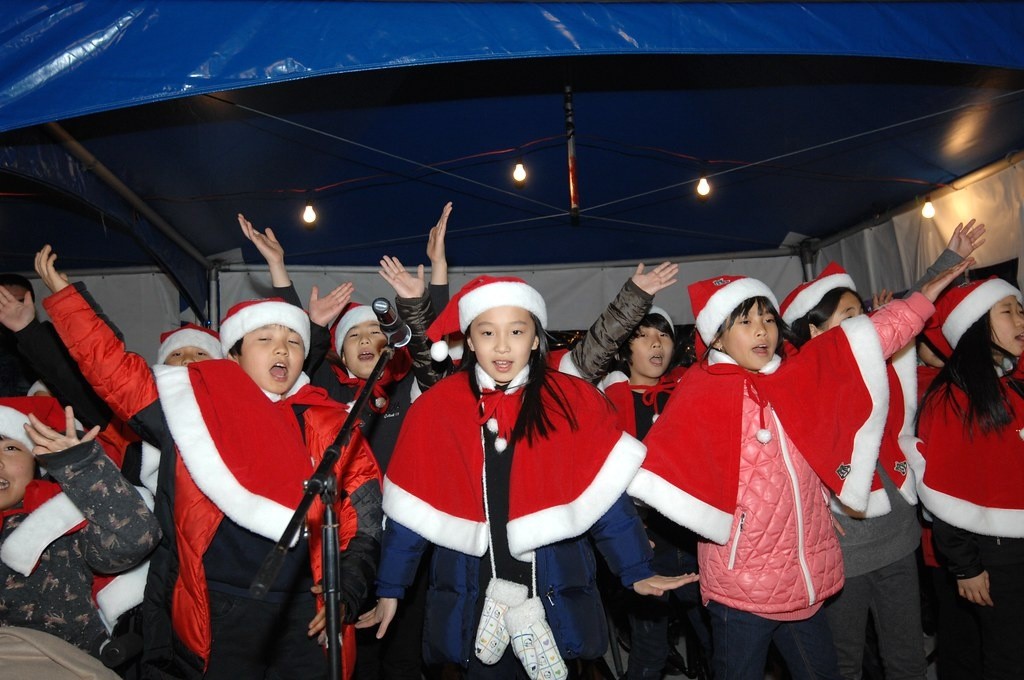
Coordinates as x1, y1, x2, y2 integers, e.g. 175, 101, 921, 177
371, 297, 412, 347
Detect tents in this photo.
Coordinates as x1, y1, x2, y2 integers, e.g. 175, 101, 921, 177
0, 0, 1024, 331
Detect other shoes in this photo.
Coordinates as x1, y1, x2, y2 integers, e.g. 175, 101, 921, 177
664, 647, 685, 675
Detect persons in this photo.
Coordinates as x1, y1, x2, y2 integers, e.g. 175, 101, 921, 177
0, 201, 1024, 680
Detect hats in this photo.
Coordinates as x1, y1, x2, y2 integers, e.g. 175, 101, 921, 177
780, 261, 857, 329
923, 274, 1024, 357
0, 396, 67, 476
157, 322, 223, 365
330, 302, 379, 359
614, 305, 675, 361
689, 275, 780, 361
219, 297, 311, 359
424, 276, 547, 362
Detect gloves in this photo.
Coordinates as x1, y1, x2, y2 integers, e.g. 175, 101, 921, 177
516, 605, 569, 680
474, 577, 528, 663
